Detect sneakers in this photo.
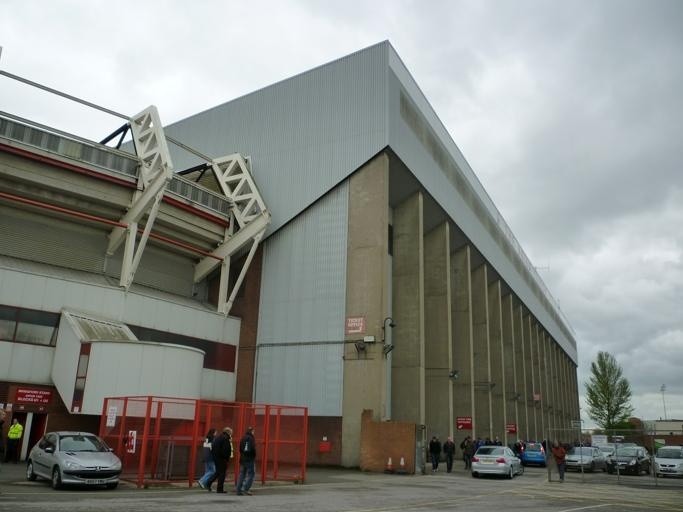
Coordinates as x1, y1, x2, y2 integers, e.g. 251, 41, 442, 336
244, 490, 252, 496
198, 480, 204, 489
216, 490, 228, 493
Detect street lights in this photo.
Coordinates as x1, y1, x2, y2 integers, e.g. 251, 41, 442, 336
659, 382, 667, 420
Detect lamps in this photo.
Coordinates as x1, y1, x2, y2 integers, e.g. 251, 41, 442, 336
380, 316, 396, 343
383, 343, 394, 355
449, 368, 461, 380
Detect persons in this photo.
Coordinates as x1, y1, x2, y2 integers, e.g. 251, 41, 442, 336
429, 436, 590, 482
234, 426, 256, 495
4, 419, 23, 464
197, 427, 233, 494
0, 409, 7, 437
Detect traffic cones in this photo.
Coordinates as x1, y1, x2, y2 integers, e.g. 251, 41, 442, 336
383, 456, 393, 474
391, 456, 409, 474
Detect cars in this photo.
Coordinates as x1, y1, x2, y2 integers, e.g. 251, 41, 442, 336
23, 426, 122, 492
603, 446, 651, 478
562, 446, 606, 475
516, 442, 547, 468
591, 442, 638, 464
468, 445, 525, 482
650, 445, 682, 479
